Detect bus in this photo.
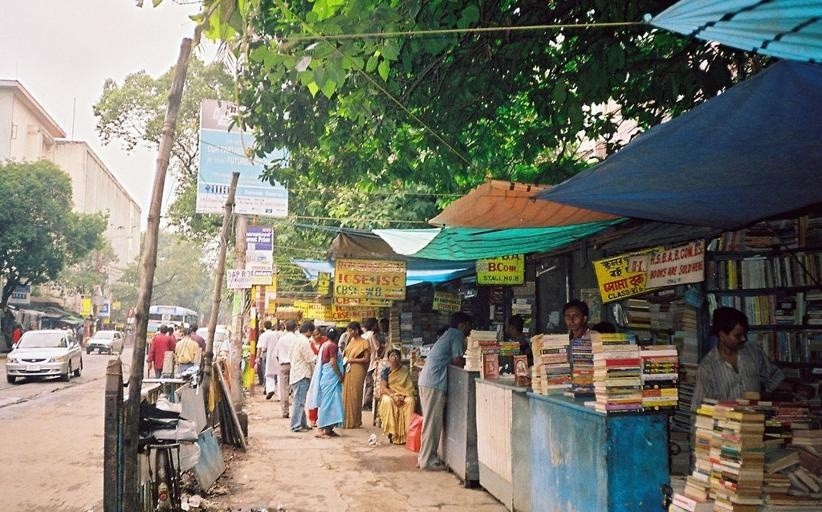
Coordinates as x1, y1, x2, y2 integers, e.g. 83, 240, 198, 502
147, 304, 198, 346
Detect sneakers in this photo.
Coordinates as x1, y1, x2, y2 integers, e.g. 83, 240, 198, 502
264, 387, 312, 432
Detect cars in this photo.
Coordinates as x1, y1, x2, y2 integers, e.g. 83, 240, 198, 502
6, 329, 85, 385
88, 330, 124, 355
194, 328, 231, 357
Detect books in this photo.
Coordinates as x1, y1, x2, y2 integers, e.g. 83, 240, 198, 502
399, 309, 435, 358
462, 323, 685, 412
607, 213, 822, 511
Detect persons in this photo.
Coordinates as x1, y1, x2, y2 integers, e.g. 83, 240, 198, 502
52, 322, 97, 345
239, 308, 389, 437
11, 325, 23, 345
504, 314, 534, 365
562, 298, 602, 343
145, 317, 213, 378
377, 348, 417, 446
416, 311, 473, 470
